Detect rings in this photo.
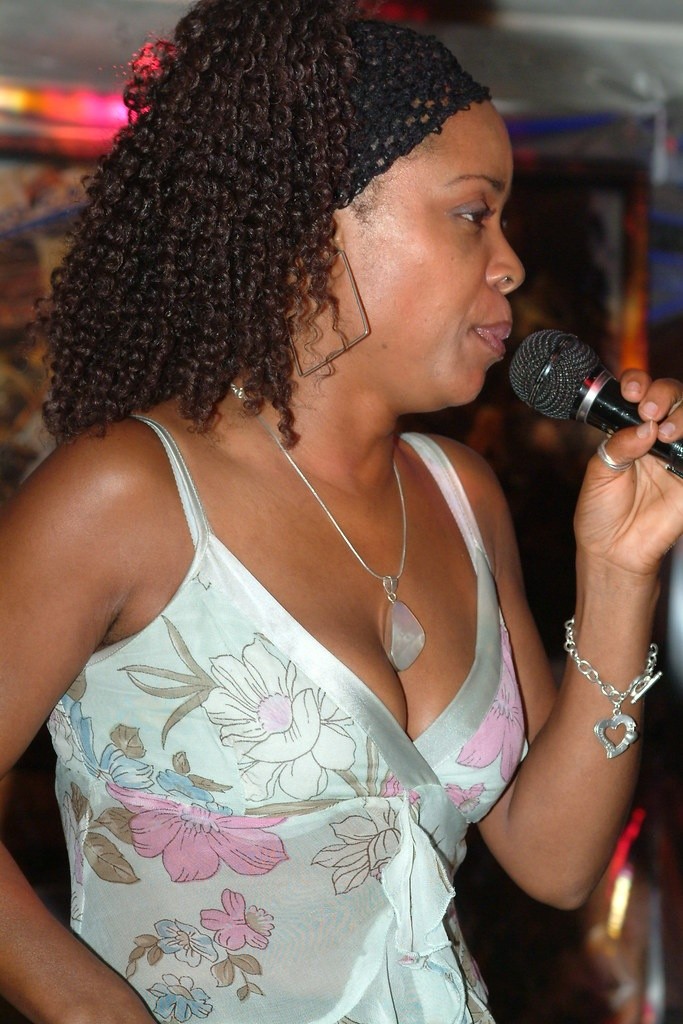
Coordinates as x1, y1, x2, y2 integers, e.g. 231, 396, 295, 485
597, 437, 634, 469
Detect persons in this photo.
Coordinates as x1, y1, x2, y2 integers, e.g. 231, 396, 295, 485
0, 0, 683, 1024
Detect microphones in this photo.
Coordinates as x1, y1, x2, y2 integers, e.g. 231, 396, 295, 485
509, 328, 683, 481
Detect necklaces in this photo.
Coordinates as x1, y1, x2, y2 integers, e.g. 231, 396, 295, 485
232, 382, 426, 671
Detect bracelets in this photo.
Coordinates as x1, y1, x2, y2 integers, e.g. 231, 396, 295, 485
564, 612, 661, 760
